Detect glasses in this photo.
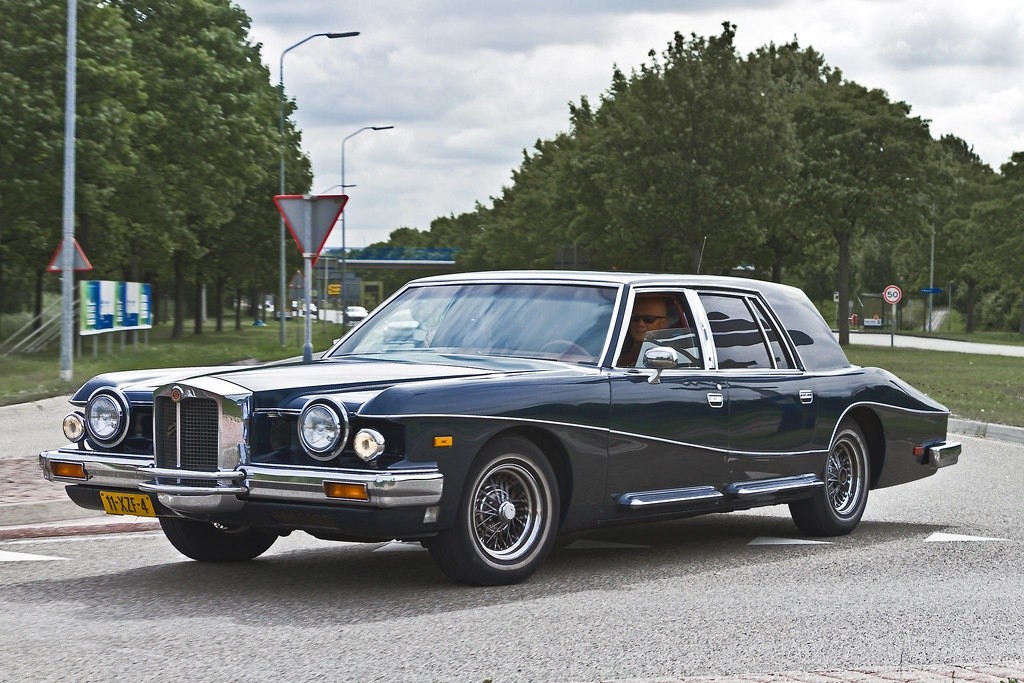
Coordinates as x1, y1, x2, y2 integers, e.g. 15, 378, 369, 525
631, 311, 679, 323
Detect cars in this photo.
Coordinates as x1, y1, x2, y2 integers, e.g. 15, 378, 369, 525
292, 300, 318, 315
38, 270, 961, 586
345, 306, 369, 323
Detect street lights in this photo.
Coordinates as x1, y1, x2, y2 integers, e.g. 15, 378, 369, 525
279, 31, 360, 352
341, 125, 395, 339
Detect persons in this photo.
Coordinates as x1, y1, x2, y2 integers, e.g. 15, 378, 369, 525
618, 296, 682, 371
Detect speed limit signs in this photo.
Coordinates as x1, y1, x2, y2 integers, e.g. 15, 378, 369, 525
882, 284, 901, 304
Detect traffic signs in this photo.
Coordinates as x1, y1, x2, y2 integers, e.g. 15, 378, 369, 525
920, 287, 943, 293
